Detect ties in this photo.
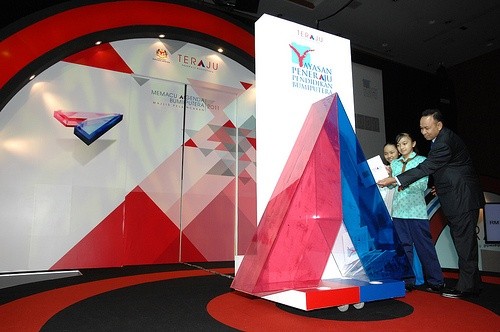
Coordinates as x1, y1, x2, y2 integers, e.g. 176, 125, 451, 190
398, 155, 417, 192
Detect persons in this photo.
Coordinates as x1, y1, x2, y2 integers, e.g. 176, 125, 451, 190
380, 144, 399, 179
390, 132, 445, 293
377, 108, 484, 298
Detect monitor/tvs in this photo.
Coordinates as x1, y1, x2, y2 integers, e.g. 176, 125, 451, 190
483, 202, 500, 246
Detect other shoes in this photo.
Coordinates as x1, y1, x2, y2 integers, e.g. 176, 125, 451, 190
426, 280, 448, 293
405, 283, 412, 292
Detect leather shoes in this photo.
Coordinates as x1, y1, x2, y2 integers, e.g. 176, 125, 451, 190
472, 288, 480, 298
441, 289, 473, 299
